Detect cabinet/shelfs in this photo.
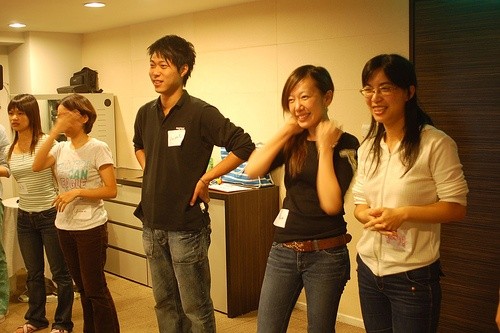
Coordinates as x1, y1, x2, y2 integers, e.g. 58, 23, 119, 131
103, 167, 280, 320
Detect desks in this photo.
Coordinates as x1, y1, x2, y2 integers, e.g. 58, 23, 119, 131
1, 197, 60, 293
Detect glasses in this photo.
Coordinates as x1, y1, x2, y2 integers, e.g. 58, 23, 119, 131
359, 86, 401, 97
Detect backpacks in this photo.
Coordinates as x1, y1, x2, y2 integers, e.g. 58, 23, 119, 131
70, 67, 104, 93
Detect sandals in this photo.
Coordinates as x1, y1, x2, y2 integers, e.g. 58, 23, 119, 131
50, 328, 68, 333
14, 323, 48, 333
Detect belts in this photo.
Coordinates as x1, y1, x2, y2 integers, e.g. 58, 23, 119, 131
282, 233, 352, 252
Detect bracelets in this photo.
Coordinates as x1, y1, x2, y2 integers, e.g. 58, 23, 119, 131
199, 178, 209, 186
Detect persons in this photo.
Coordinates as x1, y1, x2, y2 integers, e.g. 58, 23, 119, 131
132, 34, 255, 333
0, 124, 13, 320
33, 94, 120, 333
246, 64, 360, 332
4, 93, 75, 333
354, 53, 469, 333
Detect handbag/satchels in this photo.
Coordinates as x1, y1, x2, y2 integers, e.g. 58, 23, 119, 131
56, 84, 89, 93
220, 142, 274, 188
